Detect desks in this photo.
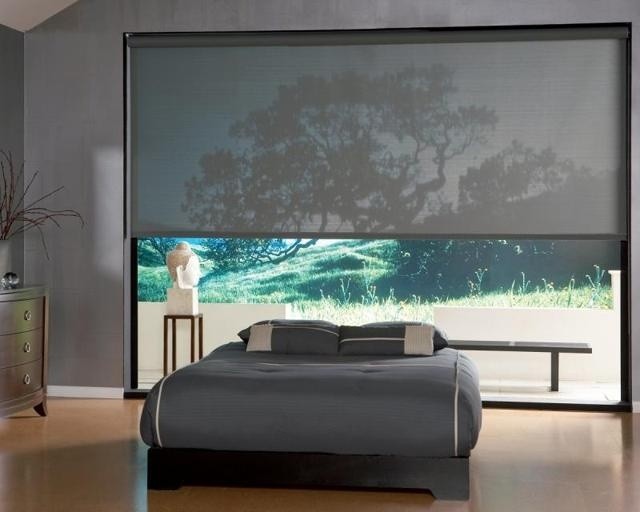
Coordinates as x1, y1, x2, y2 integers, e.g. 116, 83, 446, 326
162, 314, 204, 375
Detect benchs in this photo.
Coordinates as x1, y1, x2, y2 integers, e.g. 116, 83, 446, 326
449, 338, 593, 393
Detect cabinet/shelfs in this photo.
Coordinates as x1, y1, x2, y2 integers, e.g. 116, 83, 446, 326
1, 283, 49, 420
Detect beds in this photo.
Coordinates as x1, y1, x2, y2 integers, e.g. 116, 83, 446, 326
138, 341, 476, 502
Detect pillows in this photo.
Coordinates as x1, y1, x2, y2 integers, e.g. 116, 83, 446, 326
237, 317, 450, 357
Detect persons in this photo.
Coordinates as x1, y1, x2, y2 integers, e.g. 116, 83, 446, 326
167, 241, 203, 289
